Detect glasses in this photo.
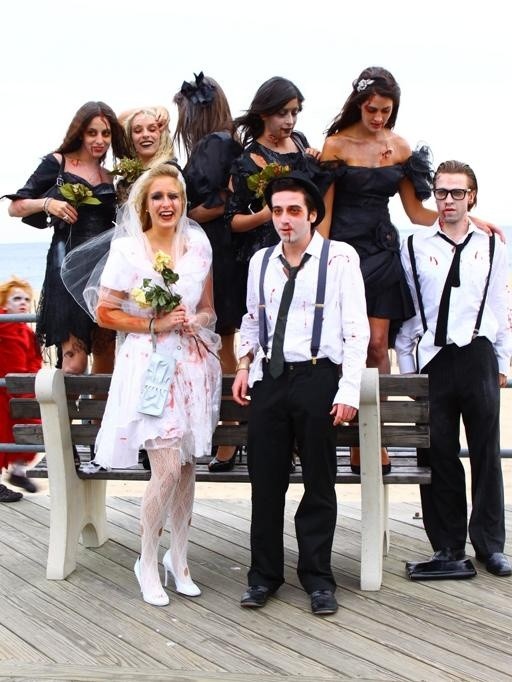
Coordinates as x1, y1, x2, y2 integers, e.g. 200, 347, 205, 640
434, 188, 473, 201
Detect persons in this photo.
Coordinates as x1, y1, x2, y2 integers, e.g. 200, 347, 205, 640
230, 169, 371, 614
60, 160, 224, 606
173, 73, 249, 471
223, 75, 322, 471
395, 161, 512, 576
0, 101, 133, 472
0, 278, 43, 502
113, 104, 173, 468
313, 67, 506, 477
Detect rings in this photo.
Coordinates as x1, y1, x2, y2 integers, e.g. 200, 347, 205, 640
63, 215, 68, 221
65, 209, 70, 214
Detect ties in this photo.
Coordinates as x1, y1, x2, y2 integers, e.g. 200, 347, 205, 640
268, 253, 312, 379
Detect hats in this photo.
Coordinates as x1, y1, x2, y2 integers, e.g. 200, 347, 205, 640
263, 170, 325, 228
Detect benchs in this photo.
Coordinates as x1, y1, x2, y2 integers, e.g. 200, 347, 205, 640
6, 369, 432, 591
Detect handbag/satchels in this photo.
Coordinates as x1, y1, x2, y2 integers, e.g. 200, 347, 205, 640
405, 559, 476, 580
135, 318, 177, 417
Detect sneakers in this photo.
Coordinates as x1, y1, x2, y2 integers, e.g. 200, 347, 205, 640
1, 485, 23, 503
10, 472, 37, 493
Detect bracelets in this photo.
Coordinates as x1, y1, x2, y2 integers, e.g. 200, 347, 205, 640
149, 317, 155, 333
44, 198, 53, 224
43, 197, 49, 213
235, 363, 249, 372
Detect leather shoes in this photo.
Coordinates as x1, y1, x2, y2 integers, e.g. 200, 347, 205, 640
310, 590, 338, 613
475, 552, 512, 576
241, 585, 271, 608
428, 546, 466, 560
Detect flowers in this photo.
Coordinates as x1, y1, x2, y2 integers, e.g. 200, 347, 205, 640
131, 250, 225, 366
106, 157, 151, 189
246, 160, 292, 200
58, 183, 103, 250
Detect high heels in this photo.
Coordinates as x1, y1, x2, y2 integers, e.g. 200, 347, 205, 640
162, 550, 202, 598
134, 556, 170, 607
382, 448, 392, 476
349, 448, 360, 474
207, 445, 242, 472
